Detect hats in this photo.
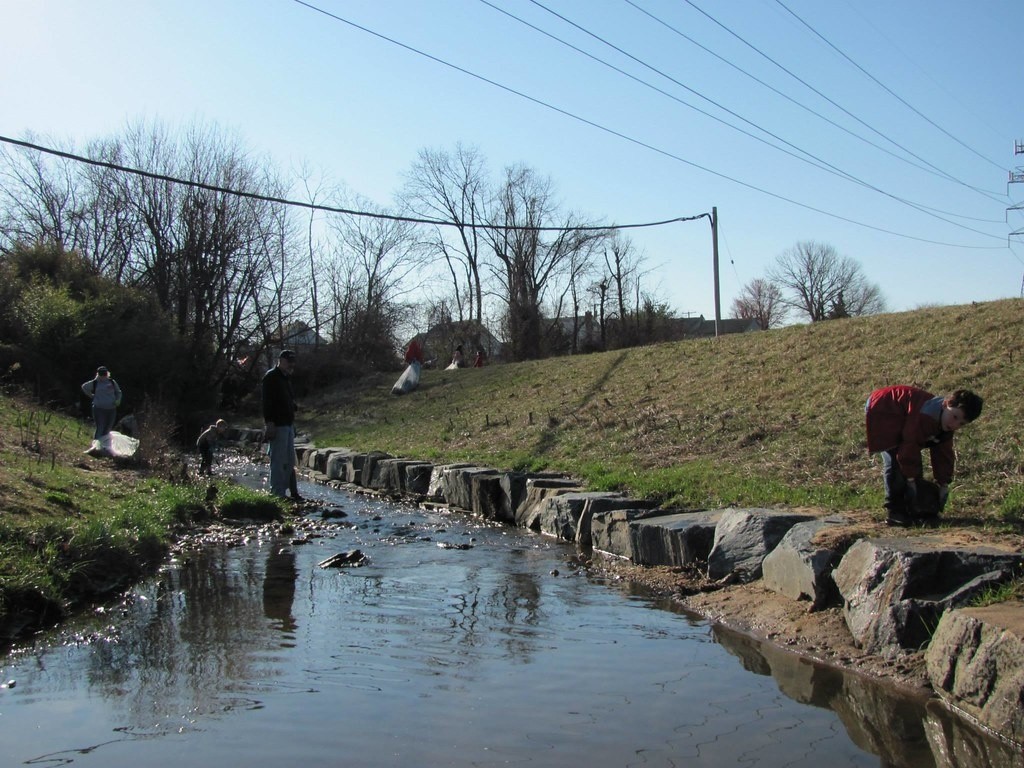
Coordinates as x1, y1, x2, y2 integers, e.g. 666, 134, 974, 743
96, 366, 108, 371
279, 349, 296, 359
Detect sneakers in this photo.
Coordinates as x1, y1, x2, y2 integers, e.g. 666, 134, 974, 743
885, 513, 909, 528
915, 511, 942, 525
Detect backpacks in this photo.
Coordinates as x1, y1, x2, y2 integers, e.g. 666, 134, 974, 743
902, 477, 946, 524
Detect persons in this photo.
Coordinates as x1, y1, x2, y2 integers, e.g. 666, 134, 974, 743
81, 366, 122, 441
452, 345, 466, 368
405, 340, 425, 366
472, 351, 483, 368
261, 351, 305, 500
866, 383, 984, 525
195, 419, 228, 476
